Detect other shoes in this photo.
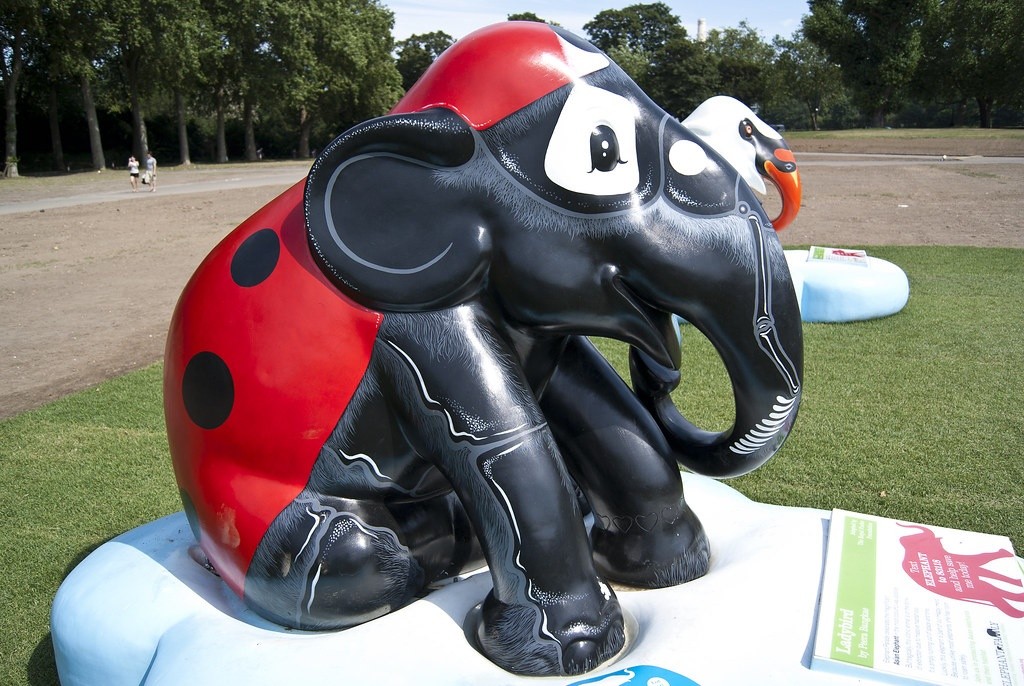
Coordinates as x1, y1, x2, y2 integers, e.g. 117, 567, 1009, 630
148, 187, 156, 192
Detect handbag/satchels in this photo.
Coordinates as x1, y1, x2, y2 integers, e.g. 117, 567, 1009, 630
140, 171, 151, 186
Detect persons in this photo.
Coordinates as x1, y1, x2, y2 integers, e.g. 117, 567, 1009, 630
128, 155, 140, 192
146, 151, 156, 192
